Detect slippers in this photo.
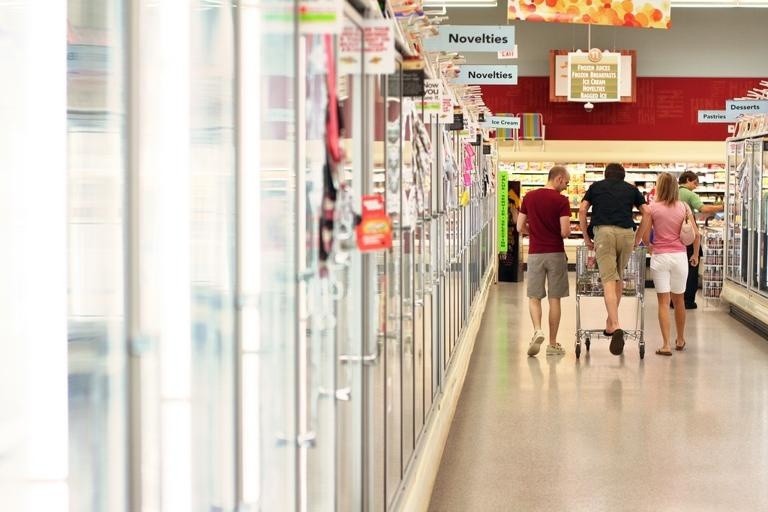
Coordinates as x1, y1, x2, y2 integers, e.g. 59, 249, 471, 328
675, 338, 686, 350
655, 348, 673, 356
603, 328, 626, 356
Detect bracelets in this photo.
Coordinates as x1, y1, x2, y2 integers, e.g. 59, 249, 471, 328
721, 204, 724, 209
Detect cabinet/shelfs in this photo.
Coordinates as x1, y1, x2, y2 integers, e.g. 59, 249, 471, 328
502, 155, 588, 271
716, 127, 768, 338
586, 160, 729, 288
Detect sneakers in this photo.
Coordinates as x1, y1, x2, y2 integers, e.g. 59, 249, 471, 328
527, 332, 545, 356
545, 342, 566, 356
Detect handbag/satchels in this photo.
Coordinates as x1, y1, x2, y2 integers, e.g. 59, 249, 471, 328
679, 216, 696, 246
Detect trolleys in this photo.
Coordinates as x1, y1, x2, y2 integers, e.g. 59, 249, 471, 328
572, 244, 648, 359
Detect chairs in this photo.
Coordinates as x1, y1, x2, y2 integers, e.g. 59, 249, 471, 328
493, 111, 519, 151
516, 111, 548, 154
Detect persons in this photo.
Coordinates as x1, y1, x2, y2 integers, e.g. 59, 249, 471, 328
515, 166, 571, 356
668, 170, 725, 309
641, 171, 700, 356
578, 162, 650, 355
